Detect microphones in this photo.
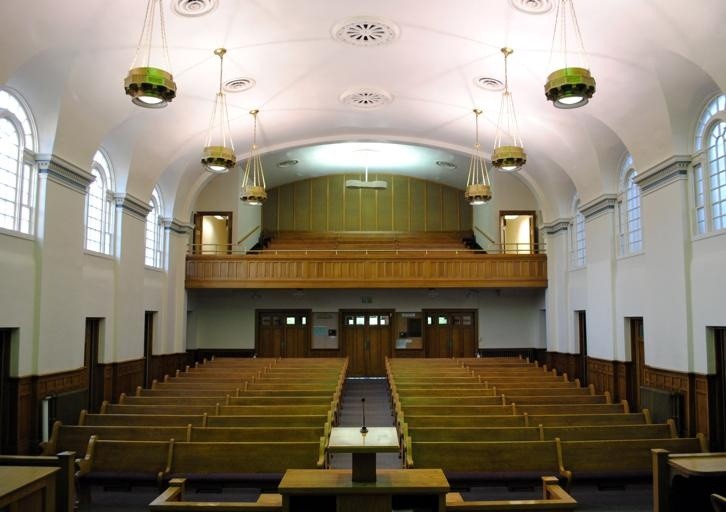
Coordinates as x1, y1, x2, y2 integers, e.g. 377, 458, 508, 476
361, 397, 368, 434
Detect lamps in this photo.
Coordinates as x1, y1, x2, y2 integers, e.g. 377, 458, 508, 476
121, 0, 268, 207
462, 0, 597, 207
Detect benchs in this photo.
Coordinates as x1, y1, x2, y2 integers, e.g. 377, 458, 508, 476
258, 230, 477, 254
